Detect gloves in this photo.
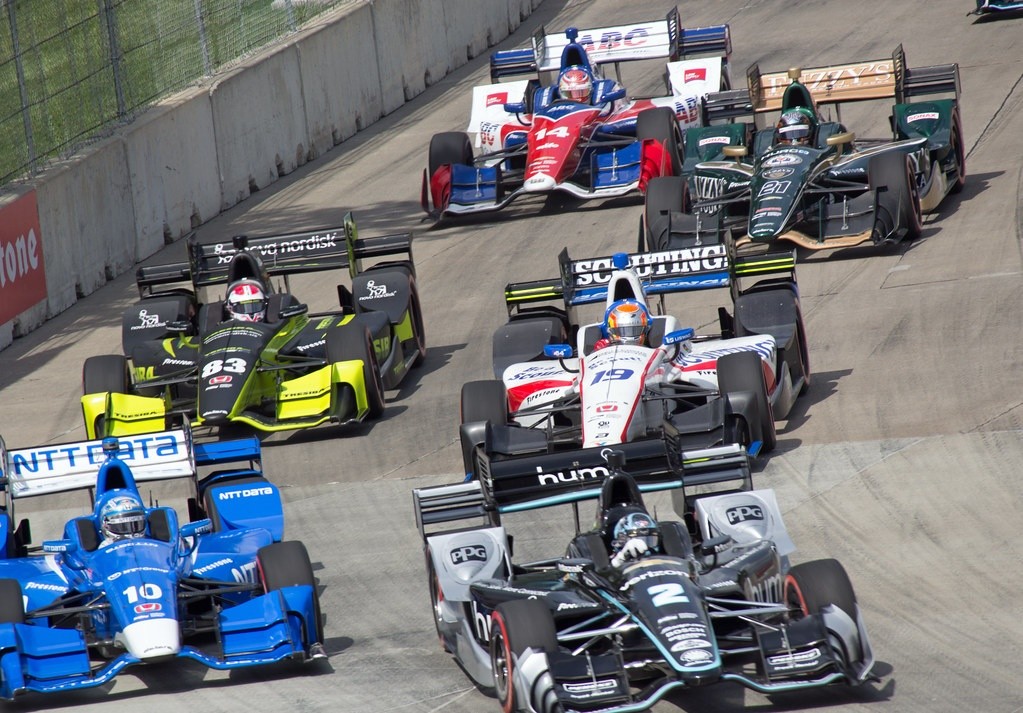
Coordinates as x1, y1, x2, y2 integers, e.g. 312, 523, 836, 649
611, 538, 651, 569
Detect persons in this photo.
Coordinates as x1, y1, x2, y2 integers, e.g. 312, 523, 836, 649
224, 279, 265, 325
595, 300, 650, 348
612, 512, 658, 566
777, 113, 813, 148
97, 496, 150, 550
557, 66, 593, 108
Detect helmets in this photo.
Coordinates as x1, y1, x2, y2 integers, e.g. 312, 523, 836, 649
99, 496, 147, 541
606, 303, 650, 346
558, 70, 594, 105
776, 111, 813, 147
225, 285, 266, 322
612, 512, 660, 557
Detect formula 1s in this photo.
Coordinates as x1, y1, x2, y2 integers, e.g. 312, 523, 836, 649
411, 411, 895, 713
637, 48, 967, 262
970, 1, 1023, 14
457, 235, 812, 482
1, 427, 330, 710
80, 208, 431, 449
420, 8, 738, 221
